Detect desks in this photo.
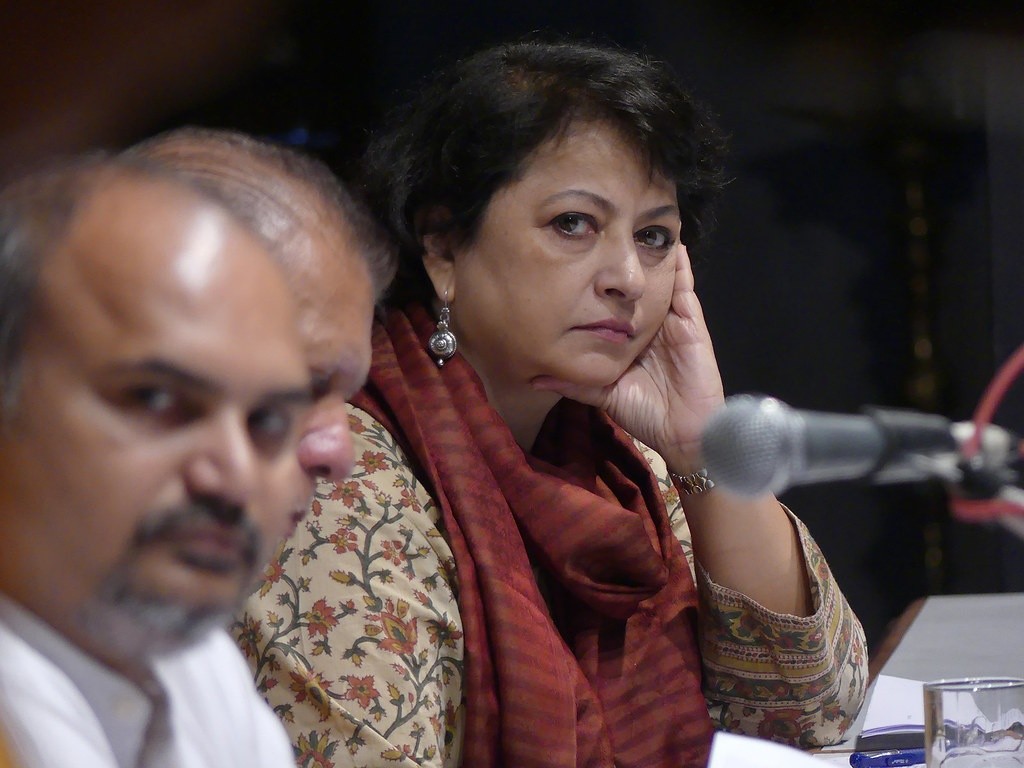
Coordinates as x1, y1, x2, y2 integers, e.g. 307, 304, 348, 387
806, 593, 1024, 768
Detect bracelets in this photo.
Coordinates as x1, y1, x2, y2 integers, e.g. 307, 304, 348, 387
667, 464, 718, 495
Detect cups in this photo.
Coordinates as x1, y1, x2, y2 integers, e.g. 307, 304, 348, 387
924, 676, 1024, 768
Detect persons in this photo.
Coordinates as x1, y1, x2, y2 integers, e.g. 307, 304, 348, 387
0, 126, 397, 768
231, 42, 868, 768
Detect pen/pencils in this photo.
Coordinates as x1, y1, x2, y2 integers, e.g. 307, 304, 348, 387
849, 739, 957, 768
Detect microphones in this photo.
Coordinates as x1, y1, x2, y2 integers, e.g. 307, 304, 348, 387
700, 391, 1018, 503
